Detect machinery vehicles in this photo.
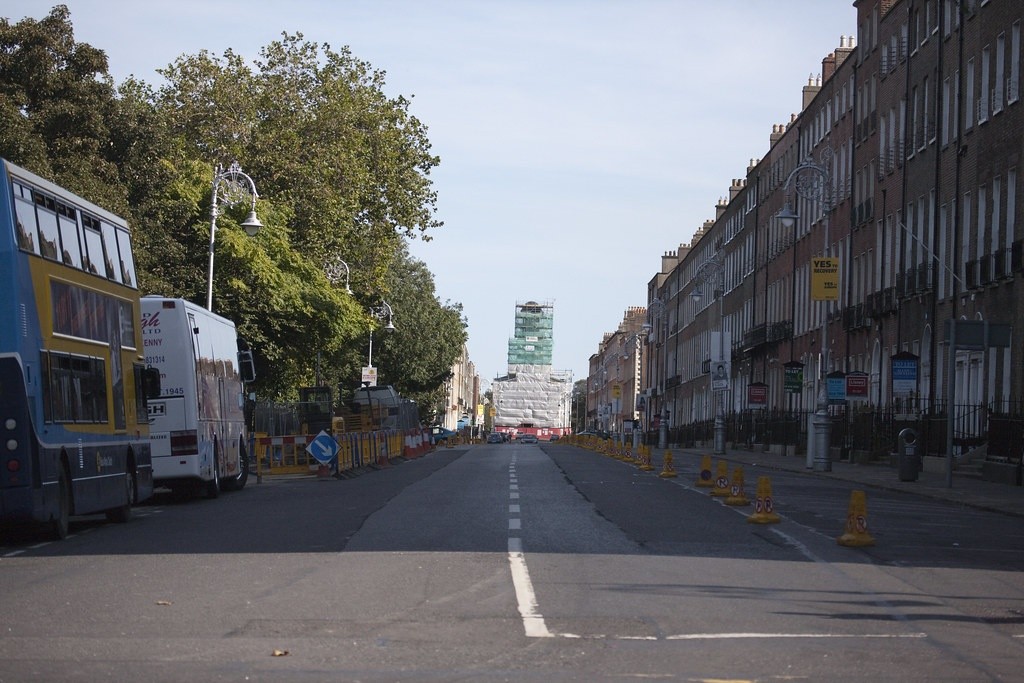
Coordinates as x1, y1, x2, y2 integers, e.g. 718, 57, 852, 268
297, 379, 403, 436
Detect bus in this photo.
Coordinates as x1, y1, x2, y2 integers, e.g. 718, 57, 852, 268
0, 155, 162, 541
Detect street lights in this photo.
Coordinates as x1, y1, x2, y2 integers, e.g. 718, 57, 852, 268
368, 300, 396, 387
689, 260, 728, 455
642, 297, 669, 449
206, 161, 265, 312
776, 143, 834, 473
316, 258, 354, 388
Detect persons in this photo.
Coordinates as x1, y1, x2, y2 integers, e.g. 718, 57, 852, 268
715, 365, 727, 380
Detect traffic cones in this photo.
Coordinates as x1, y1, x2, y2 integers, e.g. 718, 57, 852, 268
409, 428, 425, 458
429, 434, 438, 451
421, 424, 432, 454
399, 430, 416, 459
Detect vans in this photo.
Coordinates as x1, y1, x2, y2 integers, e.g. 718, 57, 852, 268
127, 293, 256, 501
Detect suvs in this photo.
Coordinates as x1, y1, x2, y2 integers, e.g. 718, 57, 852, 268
514, 431, 525, 440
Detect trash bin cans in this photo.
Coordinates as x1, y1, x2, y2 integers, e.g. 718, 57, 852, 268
898, 428, 919, 482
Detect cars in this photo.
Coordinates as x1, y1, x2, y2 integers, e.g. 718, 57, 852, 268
520, 433, 538, 444
500, 432, 510, 442
424, 426, 456, 446
577, 429, 611, 441
550, 434, 559, 443
487, 432, 503, 444
